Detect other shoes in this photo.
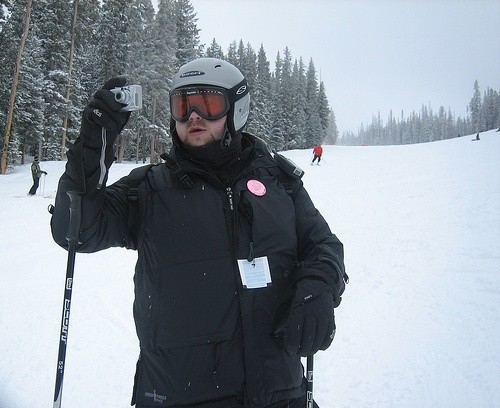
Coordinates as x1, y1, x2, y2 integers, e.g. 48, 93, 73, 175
317, 162, 320, 166
311, 161, 314, 166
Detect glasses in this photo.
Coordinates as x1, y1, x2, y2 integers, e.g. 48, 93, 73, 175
170, 87, 232, 123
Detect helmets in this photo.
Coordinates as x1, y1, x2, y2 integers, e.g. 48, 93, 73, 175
168, 56, 252, 150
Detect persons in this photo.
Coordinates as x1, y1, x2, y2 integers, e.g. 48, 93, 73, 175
29, 156, 47, 195
50, 57, 350, 408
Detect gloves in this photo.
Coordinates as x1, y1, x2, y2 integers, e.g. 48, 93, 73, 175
79, 76, 132, 150
274, 275, 335, 358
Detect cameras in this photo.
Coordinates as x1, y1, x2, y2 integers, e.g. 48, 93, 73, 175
110, 85, 142, 112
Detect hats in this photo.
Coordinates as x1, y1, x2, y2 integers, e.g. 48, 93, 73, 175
32, 154, 38, 160
